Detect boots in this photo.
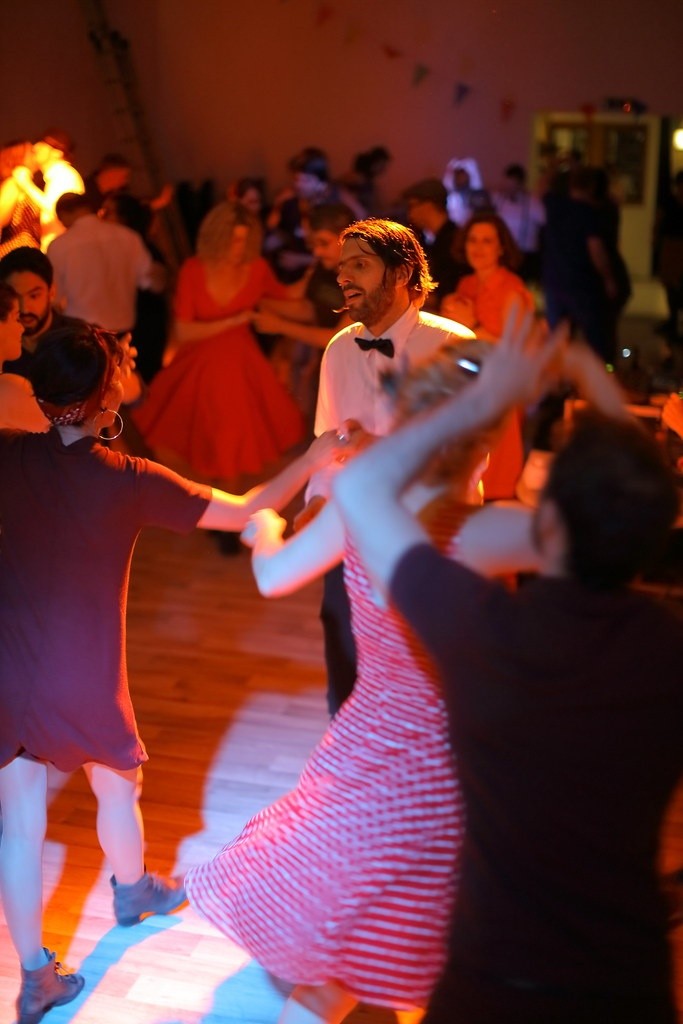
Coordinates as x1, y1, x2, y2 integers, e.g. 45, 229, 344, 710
16, 947, 84, 1024
110, 863, 187, 927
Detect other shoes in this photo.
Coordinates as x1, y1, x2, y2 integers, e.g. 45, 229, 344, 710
212, 531, 241, 556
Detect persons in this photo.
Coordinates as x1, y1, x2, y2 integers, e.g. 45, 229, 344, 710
293, 220, 490, 720
332, 300, 683, 1024
181, 340, 553, 1024
0, 316, 356, 1024
0, 134, 683, 554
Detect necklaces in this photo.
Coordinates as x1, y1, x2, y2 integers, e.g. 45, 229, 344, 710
59, 430, 85, 437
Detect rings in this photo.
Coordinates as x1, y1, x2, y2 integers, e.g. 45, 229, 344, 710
338, 434, 344, 440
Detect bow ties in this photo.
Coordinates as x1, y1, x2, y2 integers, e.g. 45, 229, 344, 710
354, 337, 394, 358
